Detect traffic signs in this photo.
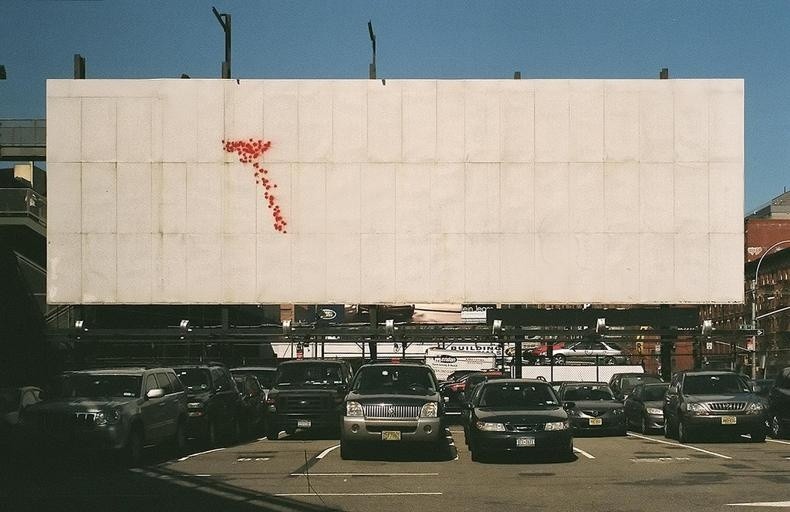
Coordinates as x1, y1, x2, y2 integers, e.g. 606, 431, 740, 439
749, 329, 764, 336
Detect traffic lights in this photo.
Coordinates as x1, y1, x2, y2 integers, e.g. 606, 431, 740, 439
746, 338, 754, 351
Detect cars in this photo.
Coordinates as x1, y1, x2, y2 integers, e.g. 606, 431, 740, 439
493, 341, 628, 366
